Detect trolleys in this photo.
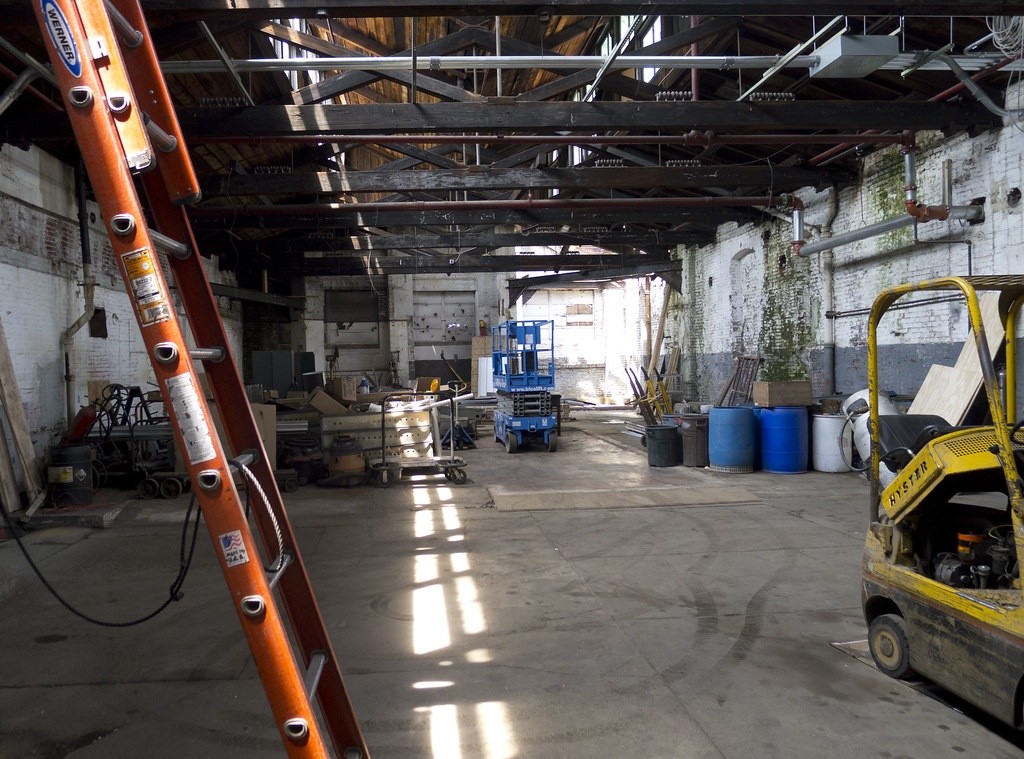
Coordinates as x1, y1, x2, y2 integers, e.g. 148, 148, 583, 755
371, 392, 467, 489
74, 381, 192, 501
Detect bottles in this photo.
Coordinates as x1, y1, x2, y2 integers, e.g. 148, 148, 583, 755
359, 378, 369, 394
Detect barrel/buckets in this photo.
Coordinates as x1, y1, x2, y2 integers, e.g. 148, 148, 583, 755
812, 413, 853, 472
709, 405, 756, 473
643, 412, 709, 468
48, 443, 93, 506
753, 407, 809, 473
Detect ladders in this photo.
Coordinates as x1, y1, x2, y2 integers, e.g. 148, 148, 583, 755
36, 0, 369, 759
